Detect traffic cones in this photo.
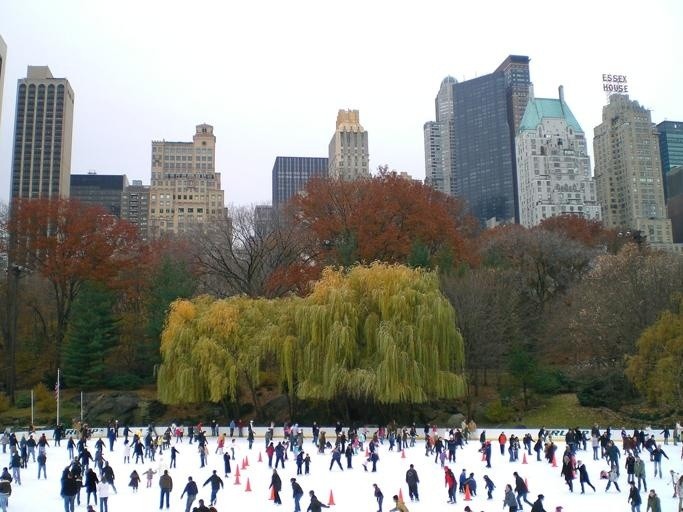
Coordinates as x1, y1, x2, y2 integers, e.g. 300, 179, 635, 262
550, 455, 558, 467
480, 453, 486, 461
326, 489, 335, 505
521, 479, 530, 492
400, 449, 406, 459
397, 489, 404, 504
521, 452, 528, 464
234, 456, 248, 486
463, 484, 471, 501
267, 489, 275, 500
257, 451, 263, 462
244, 478, 251, 491
364, 447, 369, 457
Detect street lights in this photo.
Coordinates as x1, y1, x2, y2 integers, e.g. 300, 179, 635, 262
7, 262, 33, 405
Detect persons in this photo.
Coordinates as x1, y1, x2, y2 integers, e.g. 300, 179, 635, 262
0, 419, 683, 512
